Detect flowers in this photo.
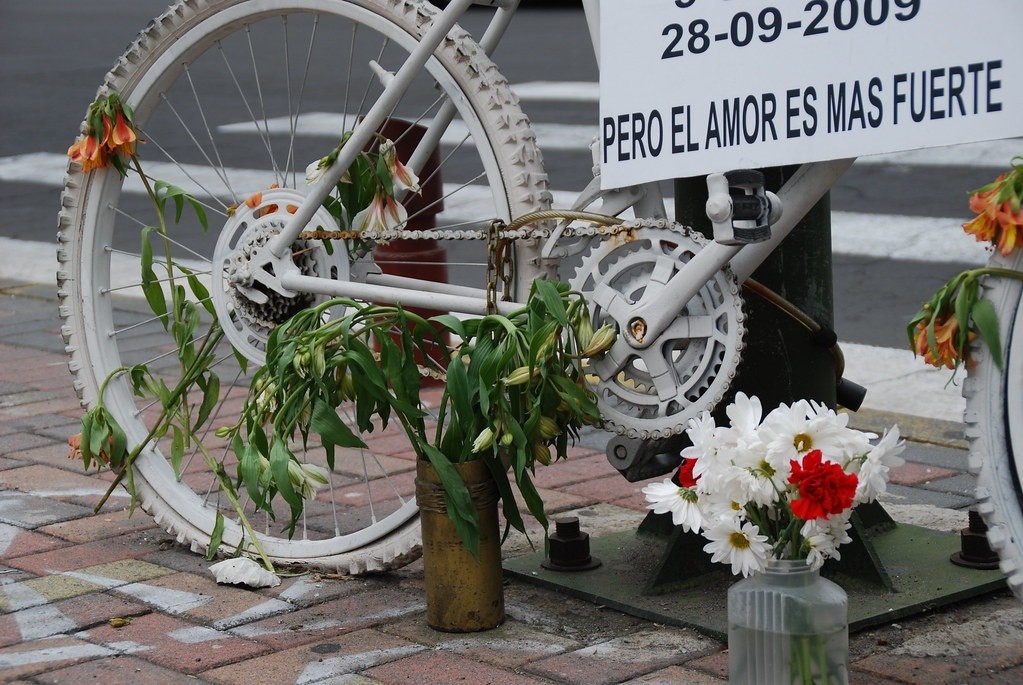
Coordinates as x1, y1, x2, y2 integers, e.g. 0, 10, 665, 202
638, 389, 906, 579
208, 271, 617, 564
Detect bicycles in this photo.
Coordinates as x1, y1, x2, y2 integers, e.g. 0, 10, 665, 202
57, 0, 1023, 599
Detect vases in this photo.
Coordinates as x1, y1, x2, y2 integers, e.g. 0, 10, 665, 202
726, 558, 849, 685
413, 450, 505, 633
